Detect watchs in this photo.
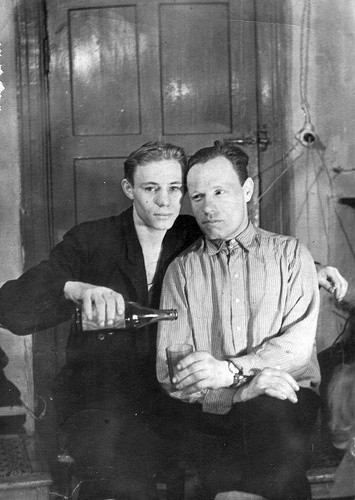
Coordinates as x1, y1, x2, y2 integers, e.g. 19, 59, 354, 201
227, 359, 241, 388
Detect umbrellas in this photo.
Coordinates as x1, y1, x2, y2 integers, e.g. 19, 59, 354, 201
0, 346, 48, 422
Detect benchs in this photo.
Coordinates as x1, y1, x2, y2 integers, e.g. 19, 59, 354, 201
1, 432, 343, 499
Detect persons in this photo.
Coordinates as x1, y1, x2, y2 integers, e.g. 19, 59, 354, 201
157, 145, 320, 500
0, 140, 348, 500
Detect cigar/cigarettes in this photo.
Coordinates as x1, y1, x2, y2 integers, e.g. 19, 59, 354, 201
333, 283, 337, 290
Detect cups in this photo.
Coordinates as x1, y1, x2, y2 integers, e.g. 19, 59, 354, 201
166, 344, 194, 389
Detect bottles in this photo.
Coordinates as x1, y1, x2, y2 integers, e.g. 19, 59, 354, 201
76, 301, 179, 334
